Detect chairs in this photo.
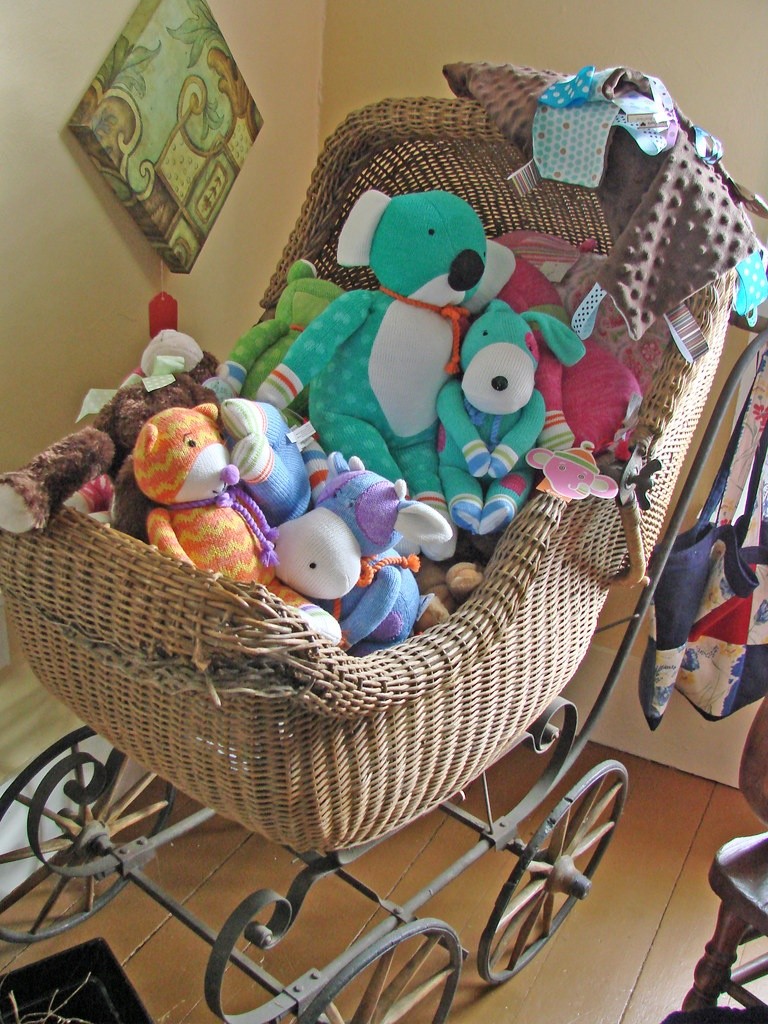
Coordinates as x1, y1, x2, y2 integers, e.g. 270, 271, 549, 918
678, 702, 768, 1024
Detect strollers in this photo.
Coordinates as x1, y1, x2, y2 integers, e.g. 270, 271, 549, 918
0, 90, 768, 1024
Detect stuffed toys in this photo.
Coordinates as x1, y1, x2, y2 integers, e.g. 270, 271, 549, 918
0, 188, 648, 663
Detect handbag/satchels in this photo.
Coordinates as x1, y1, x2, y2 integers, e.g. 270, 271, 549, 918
639, 356, 767, 729
